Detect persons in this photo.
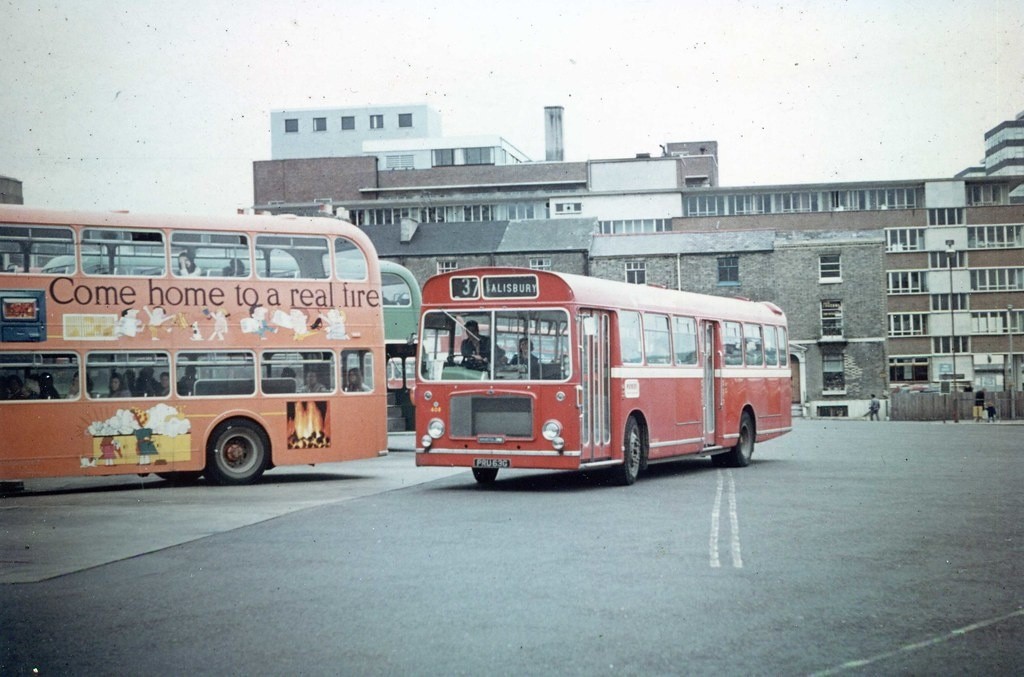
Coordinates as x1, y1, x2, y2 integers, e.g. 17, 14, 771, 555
985, 402, 997, 423
108, 373, 132, 397
870, 394, 880, 421
302, 371, 328, 392
346, 367, 371, 392
222, 258, 245, 277
973, 388, 985, 423
179, 253, 202, 276
123, 366, 197, 396
0, 371, 93, 400
510, 337, 539, 380
460, 320, 505, 366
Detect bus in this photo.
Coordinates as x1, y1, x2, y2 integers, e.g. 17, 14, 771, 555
0, 204, 388, 486
33, 252, 422, 436
410, 266, 793, 485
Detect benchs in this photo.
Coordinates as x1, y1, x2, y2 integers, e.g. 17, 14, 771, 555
193, 378, 296, 396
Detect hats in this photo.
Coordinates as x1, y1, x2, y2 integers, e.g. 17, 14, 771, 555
34, 373, 53, 383
465, 321, 478, 328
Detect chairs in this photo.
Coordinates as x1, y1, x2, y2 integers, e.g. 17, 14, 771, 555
537, 362, 566, 379
6, 263, 299, 278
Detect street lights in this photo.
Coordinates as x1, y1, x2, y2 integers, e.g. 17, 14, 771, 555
1006, 304, 1016, 421
946, 248, 960, 423
